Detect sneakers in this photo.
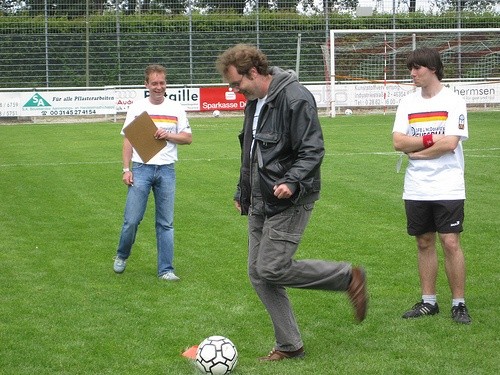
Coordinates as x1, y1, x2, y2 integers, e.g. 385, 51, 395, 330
348, 266, 366, 320
402, 299, 440, 319
451, 302, 471, 325
260, 346, 305, 362
161, 272, 180, 280
112, 256, 126, 272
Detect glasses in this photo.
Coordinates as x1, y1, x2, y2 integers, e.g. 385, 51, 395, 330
229, 74, 245, 88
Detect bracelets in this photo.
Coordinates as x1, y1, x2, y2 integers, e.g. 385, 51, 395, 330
122, 168, 130, 174
422, 134, 434, 148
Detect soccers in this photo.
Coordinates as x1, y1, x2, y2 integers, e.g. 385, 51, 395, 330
196, 335, 238, 375
345, 109, 352, 115
213, 110, 220, 117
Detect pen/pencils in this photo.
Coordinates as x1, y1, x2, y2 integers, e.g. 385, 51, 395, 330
129, 182, 133, 187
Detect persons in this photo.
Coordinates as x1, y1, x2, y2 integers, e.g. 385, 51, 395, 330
216, 41, 368, 362
391, 45, 472, 326
112, 65, 192, 281
